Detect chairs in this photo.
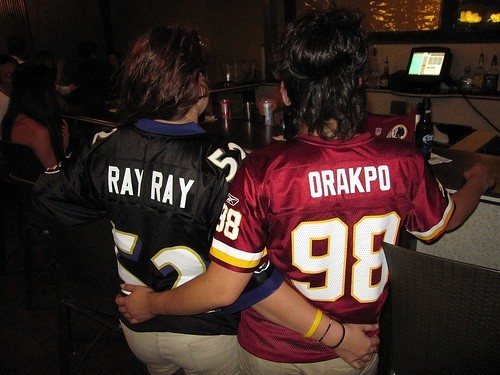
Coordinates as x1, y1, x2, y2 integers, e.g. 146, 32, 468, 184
380, 241, 500, 375
0, 139, 123, 375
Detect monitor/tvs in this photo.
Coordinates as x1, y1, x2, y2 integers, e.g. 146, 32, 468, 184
402, 46, 456, 94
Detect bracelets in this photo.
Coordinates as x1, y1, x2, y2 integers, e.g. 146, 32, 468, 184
306, 309, 345, 348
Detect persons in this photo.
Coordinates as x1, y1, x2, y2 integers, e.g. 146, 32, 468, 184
19, 25, 381, 375
0, 34, 138, 230
115, 8, 496, 375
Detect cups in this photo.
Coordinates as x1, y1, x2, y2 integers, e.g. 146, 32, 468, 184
224, 61, 257, 88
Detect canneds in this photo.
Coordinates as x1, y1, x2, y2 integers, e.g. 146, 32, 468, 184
219, 98, 231, 119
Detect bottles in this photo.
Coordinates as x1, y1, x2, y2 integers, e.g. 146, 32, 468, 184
482, 56, 499, 94
367, 48, 379, 89
415, 98, 433, 163
380, 56, 390, 89
472, 53, 485, 95
459, 67, 472, 94
